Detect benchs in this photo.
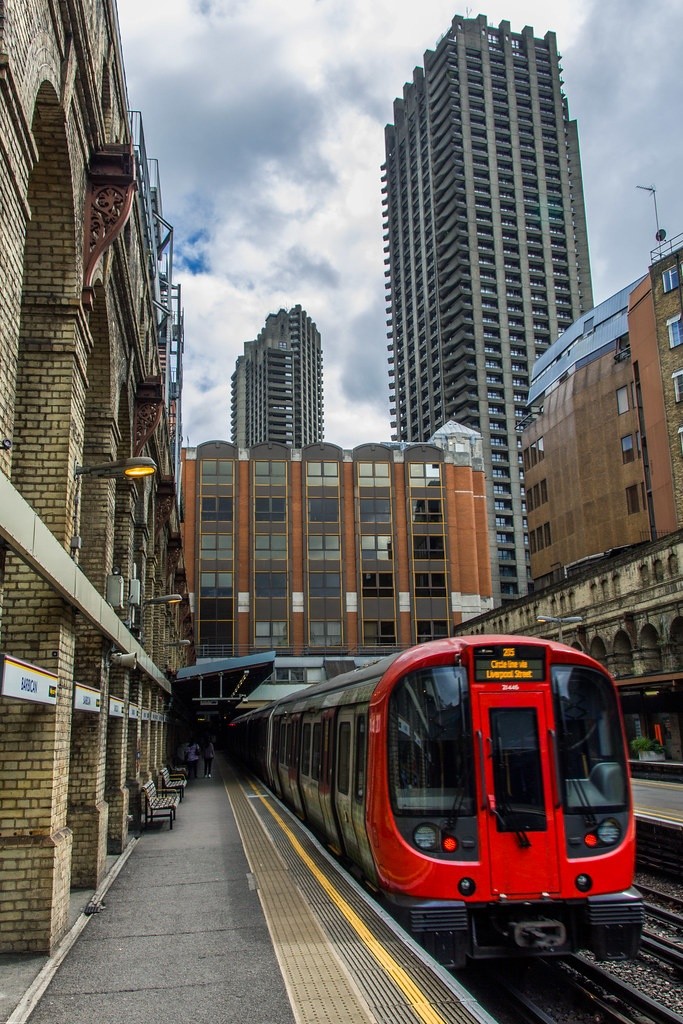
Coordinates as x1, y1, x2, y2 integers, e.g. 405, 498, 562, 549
140, 780, 181, 830
160, 761, 190, 803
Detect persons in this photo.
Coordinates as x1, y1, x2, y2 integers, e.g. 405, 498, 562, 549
185, 740, 201, 779
202, 739, 215, 778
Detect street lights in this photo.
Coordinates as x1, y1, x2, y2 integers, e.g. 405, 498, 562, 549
537, 616, 583, 645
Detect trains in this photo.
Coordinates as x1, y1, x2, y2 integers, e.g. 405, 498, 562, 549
226, 635, 646, 973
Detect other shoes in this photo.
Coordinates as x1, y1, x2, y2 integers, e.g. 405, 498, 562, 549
195, 776, 197, 779
188, 776, 191, 780
208, 774, 212, 777
204, 775, 208, 778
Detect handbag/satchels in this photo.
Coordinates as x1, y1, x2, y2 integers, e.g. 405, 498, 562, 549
195, 744, 202, 756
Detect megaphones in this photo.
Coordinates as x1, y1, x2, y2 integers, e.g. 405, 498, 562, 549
111, 652, 137, 670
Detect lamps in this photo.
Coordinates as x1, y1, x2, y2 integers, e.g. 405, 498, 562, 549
145, 592, 183, 604
76, 455, 159, 482
165, 638, 191, 648
